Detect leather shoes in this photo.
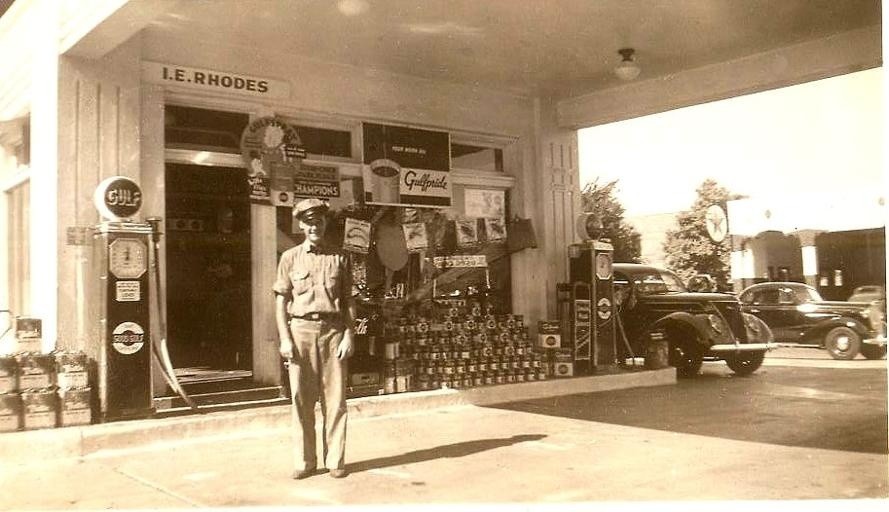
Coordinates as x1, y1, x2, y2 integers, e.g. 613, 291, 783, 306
293, 466, 317, 479
329, 470, 345, 478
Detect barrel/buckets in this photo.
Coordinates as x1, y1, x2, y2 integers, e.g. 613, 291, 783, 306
369, 158, 403, 204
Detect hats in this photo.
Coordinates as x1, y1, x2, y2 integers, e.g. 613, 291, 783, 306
292, 199, 329, 221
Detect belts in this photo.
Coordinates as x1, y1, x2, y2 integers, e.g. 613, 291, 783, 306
293, 312, 324, 321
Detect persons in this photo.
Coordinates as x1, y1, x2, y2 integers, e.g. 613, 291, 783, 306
273, 197, 358, 480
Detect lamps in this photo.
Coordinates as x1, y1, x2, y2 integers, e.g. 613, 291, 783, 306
614, 48, 641, 81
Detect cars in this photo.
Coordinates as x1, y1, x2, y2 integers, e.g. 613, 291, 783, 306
738, 281, 886, 360
612, 261, 778, 379
